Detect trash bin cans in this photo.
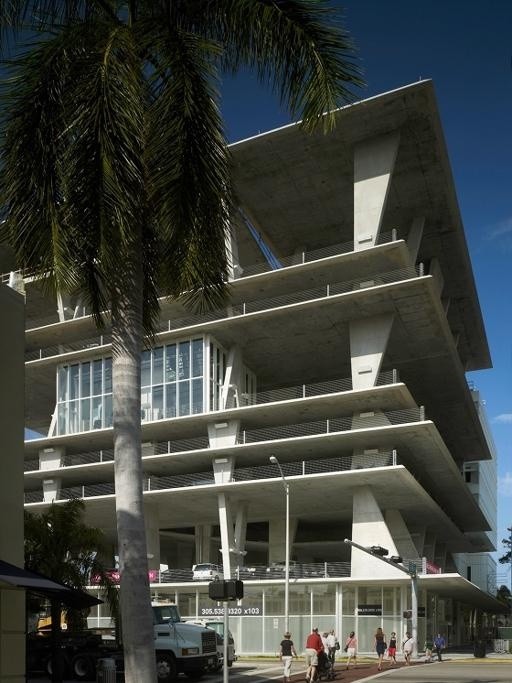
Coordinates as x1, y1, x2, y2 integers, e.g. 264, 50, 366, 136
473, 638, 487, 657
95, 658, 117, 683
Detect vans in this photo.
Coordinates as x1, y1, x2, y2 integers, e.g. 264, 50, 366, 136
184, 619, 237, 667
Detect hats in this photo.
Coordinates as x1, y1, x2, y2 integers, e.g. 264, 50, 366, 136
284, 632, 292, 636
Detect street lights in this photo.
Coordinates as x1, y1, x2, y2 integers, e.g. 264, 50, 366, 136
269, 456, 290, 633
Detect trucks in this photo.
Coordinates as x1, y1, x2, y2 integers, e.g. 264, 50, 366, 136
35, 600, 218, 683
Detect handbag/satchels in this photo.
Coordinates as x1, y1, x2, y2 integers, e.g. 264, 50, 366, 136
344, 639, 352, 652
335, 642, 341, 650
383, 642, 387, 649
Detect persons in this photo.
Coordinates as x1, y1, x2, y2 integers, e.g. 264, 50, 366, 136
325, 628, 337, 671
388, 631, 398, 665
371, 627, 387, 671
343, 631, 359, 670
278, 631, 298, 682
433, 632, 446, 662
302, 627, 323, 682
402, 631, 415, 665
321, 632, 330, 659
422, 633, 435, 664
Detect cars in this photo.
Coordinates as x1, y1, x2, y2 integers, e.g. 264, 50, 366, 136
154, 561, 350, 583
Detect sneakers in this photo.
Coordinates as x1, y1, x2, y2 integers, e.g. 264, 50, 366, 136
304, 676, 315, 683
347, 667, 348, 670
